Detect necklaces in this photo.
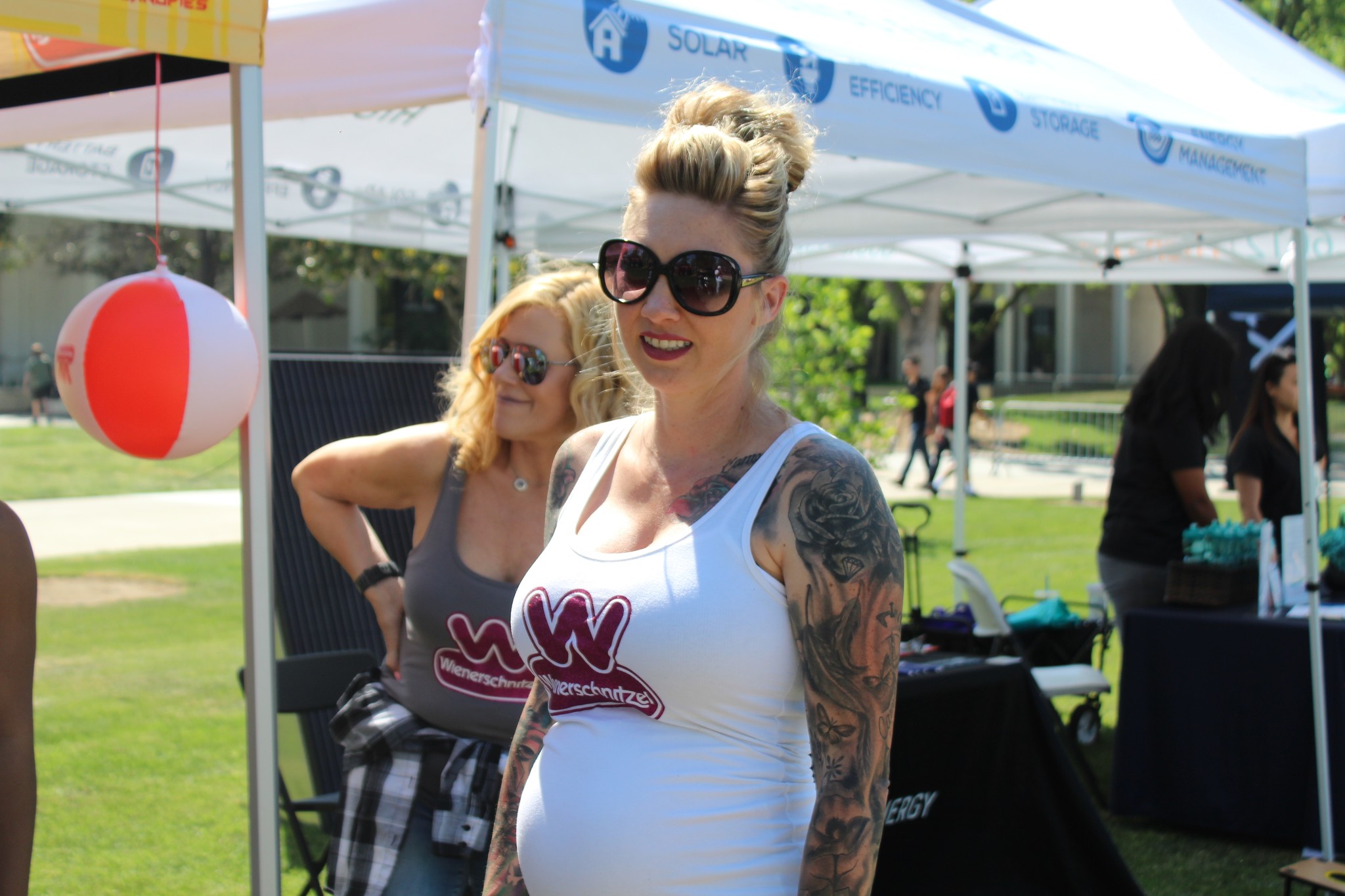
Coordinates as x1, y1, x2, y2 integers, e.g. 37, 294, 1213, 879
506, 458, 550, 490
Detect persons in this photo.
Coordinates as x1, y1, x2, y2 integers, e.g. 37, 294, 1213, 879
1096, 305, 1252, 810
292, 265, 657, 896
1226, 351, 1327, 575
889, 354, 982, 499
483, 80, 903, 896
0, 501, 38, 896
23, 343, 58, 426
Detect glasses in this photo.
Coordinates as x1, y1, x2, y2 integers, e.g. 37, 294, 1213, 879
479, 336, 578, 385
596, 239, 772, 317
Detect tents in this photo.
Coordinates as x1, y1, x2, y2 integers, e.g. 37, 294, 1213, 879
1153, 284, 1345, 341
0, 0, 1345, 862
0, 0, 282, 896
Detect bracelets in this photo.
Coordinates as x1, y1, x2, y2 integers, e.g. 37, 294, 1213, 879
353, 563, 403, 596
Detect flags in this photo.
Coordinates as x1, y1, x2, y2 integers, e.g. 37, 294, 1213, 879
1211, 310, 1330, 490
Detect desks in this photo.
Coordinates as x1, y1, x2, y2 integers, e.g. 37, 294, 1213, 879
902, 614, 1098, 666
870, 653, 1145, 896
1107, 603, 1344, 858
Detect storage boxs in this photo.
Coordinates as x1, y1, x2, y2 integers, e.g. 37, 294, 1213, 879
1170, 557, 1256, 608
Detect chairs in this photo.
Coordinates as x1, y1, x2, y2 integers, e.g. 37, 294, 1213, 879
237, 649, 382, 896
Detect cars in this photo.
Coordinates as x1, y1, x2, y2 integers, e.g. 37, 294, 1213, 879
1324, 371, 1345, 399
